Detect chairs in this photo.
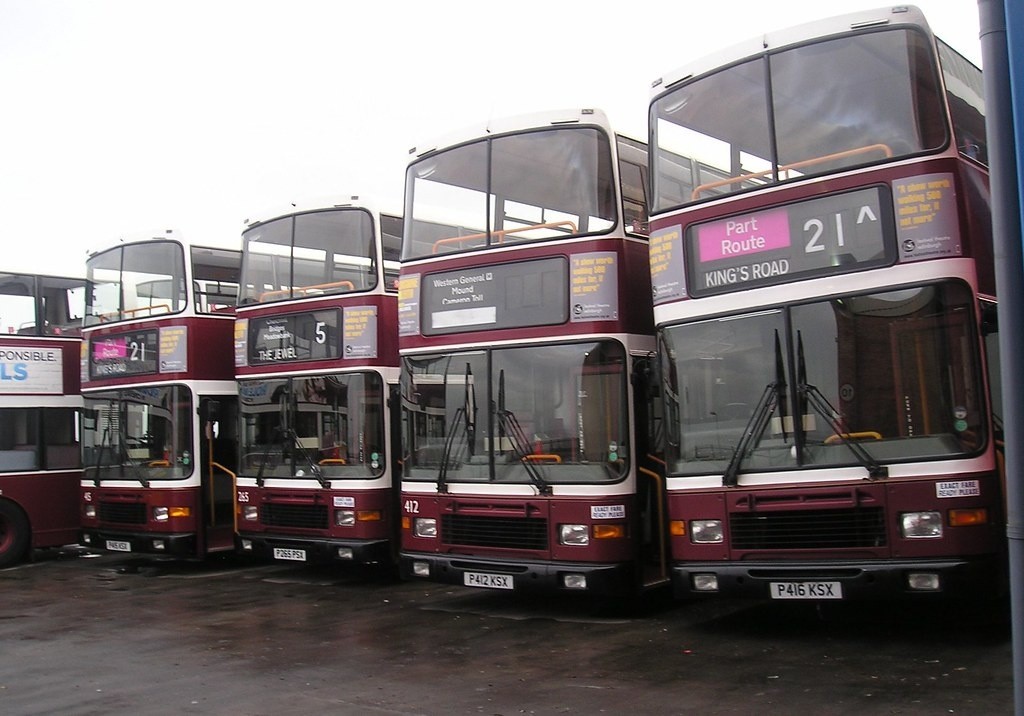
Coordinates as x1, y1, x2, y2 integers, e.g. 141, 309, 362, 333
18, 321, 37, 336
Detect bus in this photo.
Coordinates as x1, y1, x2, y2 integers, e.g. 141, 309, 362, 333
227, 197, 556, 587
74, 230, 404, 568
386, 104, 792, 611
0, 270, 234, 572
631, 1, 1010, 638
